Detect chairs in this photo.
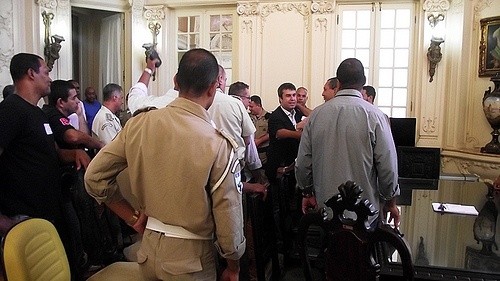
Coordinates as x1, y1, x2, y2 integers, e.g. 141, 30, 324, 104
0, 218, 72, 281
298, 180, 414, 281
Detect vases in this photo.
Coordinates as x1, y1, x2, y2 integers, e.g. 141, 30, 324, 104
473, 180, 498, 254
480, 73, 500, 155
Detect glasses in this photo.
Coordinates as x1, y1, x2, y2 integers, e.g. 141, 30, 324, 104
239, 95, 251, 100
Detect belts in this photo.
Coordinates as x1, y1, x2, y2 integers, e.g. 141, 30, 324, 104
145, 216, 214, 240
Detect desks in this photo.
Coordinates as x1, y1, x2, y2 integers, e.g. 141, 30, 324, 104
385, 176, 500, 274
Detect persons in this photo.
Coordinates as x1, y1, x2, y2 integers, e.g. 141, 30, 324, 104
84, 48, 246, 281
208, 65, 340, 265
361, 86, 376, 105
0, 53, 178, 281
294, 58, 400, 231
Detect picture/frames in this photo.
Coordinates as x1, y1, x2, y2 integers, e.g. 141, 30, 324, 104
478, 15, 500, 77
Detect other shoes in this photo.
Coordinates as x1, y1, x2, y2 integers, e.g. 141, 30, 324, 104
86, 264, 105, 277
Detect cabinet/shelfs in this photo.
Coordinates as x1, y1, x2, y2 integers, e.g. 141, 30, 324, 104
464, 247, 499, 275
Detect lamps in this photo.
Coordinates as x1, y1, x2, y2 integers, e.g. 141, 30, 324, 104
426, 25, 446, 84
41, 11, 64, 72
141, 22, 161, 81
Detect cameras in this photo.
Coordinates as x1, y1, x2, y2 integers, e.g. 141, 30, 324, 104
146, 49, 162, 67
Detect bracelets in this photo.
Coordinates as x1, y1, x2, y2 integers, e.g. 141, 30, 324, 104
145, 67, 152, 76
127, 210, 140, 226
283, 166, 290, 175
